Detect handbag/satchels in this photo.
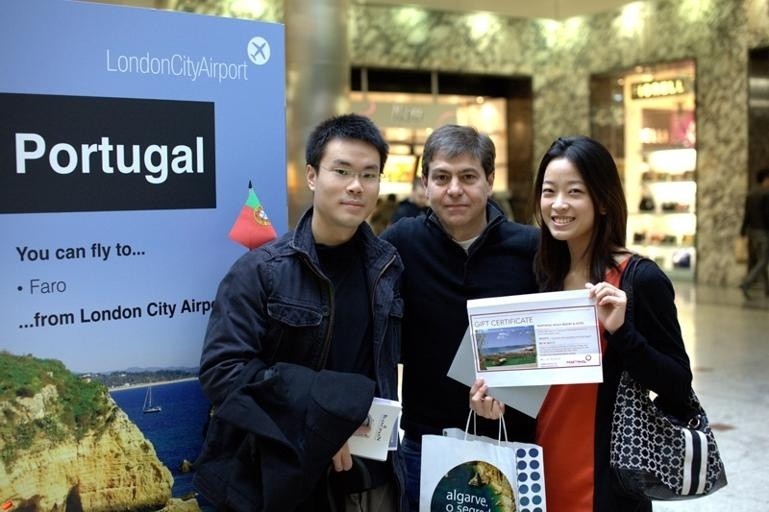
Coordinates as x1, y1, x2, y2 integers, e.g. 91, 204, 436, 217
192, 356, 266, 510
420, 398, 546, 512
609, 367, 726, 499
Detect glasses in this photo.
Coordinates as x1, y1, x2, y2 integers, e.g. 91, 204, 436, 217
316, 165, 385, 185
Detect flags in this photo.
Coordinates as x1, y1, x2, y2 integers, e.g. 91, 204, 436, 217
226, 187, 277, 249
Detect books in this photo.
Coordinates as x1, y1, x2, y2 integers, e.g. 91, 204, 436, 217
347, 397, 402, 462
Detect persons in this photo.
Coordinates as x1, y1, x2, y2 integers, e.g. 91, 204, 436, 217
191, 115, 404, 512
377, 124, 542, 511
392, 177, 429, 223
740, 170, 769, 301
468, 133, 728, 512
